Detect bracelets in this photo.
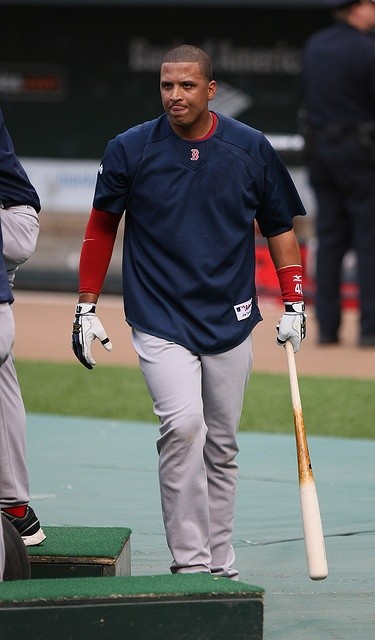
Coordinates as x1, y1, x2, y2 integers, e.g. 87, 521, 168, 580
275, 265, 304, 304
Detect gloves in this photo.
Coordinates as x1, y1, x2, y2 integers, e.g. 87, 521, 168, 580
71, 302, 112, 370
275, 301, 308, 354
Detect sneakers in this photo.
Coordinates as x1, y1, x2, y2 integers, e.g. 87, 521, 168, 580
0, 506, 46, 546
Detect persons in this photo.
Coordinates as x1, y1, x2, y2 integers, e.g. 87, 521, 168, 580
301, 1, 374, 354
1, 115, 48, 552
69, 44, 311, 585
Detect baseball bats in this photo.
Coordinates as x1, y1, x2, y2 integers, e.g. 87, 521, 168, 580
275, 322, 330, 582
